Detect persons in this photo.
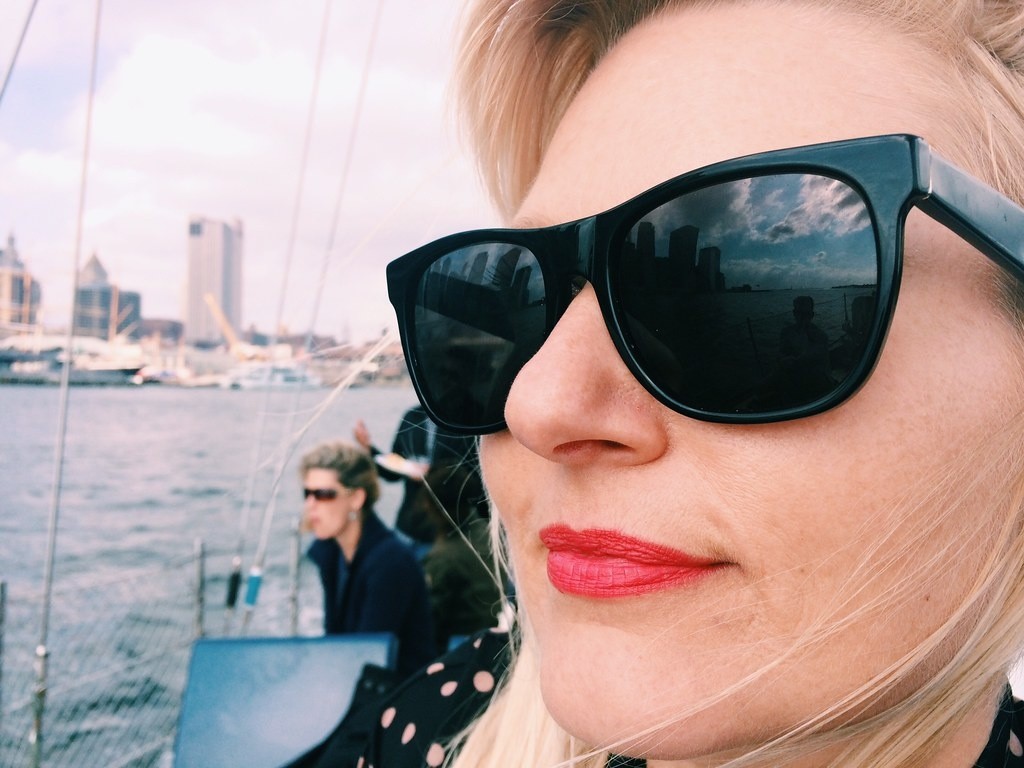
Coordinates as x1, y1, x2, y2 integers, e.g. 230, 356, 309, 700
318, 0, 1024, 768
301, 444, 444, 691
354, 406, 490, 566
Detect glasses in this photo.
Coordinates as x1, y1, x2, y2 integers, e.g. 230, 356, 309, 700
385, 132, 1024, 436
303, 487, 336, 502
